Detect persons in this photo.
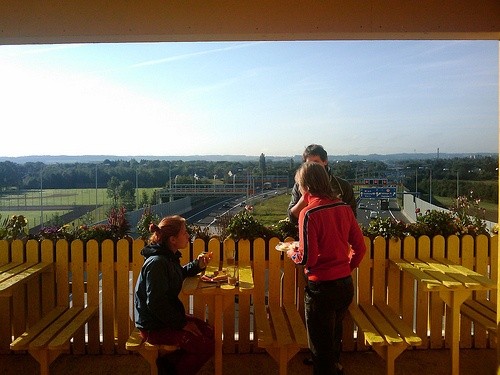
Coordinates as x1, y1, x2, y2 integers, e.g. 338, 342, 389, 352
286, 162, 366, 375
287, 143, 357, 372
136, 215, 214, 375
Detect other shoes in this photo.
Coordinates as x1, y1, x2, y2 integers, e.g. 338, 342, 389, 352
157, 357, 175, 375
303, 358, 313, 365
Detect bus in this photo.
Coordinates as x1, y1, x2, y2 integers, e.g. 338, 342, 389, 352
264, 182, 272, 190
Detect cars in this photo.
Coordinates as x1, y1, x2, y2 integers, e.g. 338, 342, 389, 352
240, 201, 247, 207
223, 202, 230, 208
358, 203, 366, 209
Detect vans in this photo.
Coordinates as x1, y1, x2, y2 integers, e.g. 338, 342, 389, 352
369, 211, 378, 219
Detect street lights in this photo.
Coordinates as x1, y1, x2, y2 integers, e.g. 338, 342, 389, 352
418, 166, 432, 204
135, 164, 147, 189
168, 164, 180, 189
243, 168, 249, 199
406, 166, 418, 197
40, 166, 53, 228
355, 159, 367, 182
96, 163, 111, 209
442, 168, 460, 209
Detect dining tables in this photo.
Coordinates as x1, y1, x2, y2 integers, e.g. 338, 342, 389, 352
182, 265, 254, 375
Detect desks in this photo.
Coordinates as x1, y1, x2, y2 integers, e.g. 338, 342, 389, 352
390, 257, 498, 375
0, 262, 52, 291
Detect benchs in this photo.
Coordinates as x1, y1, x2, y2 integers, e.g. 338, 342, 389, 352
350, 301, 422, 375
9, 304, 97, 375
459, 297, 500, 375
125, 326, 190, 375
253, 303, 310, 375
